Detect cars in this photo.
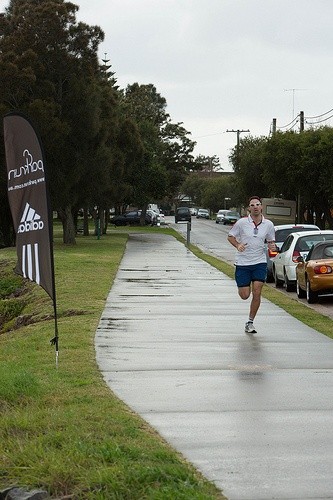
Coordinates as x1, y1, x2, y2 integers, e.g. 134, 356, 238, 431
139, 204, 160, 224
189, 208, 198, 215
216, 209, 240, 224
111, 211, 151, 225
197, 208, 210, 219
175, 207, 191, 223
266, 222, 320, 282
295, 241, 333, 305
271, 230, 333, 293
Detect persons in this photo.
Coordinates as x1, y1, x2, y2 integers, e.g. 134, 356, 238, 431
227, 196, 277, 333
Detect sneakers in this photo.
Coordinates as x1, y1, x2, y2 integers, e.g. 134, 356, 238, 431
244, 321, 257, 333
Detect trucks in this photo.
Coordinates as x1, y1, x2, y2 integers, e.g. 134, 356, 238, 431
261, 198, 296, 225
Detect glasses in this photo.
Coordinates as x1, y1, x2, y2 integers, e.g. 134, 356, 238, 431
250, 203, 262, 207
